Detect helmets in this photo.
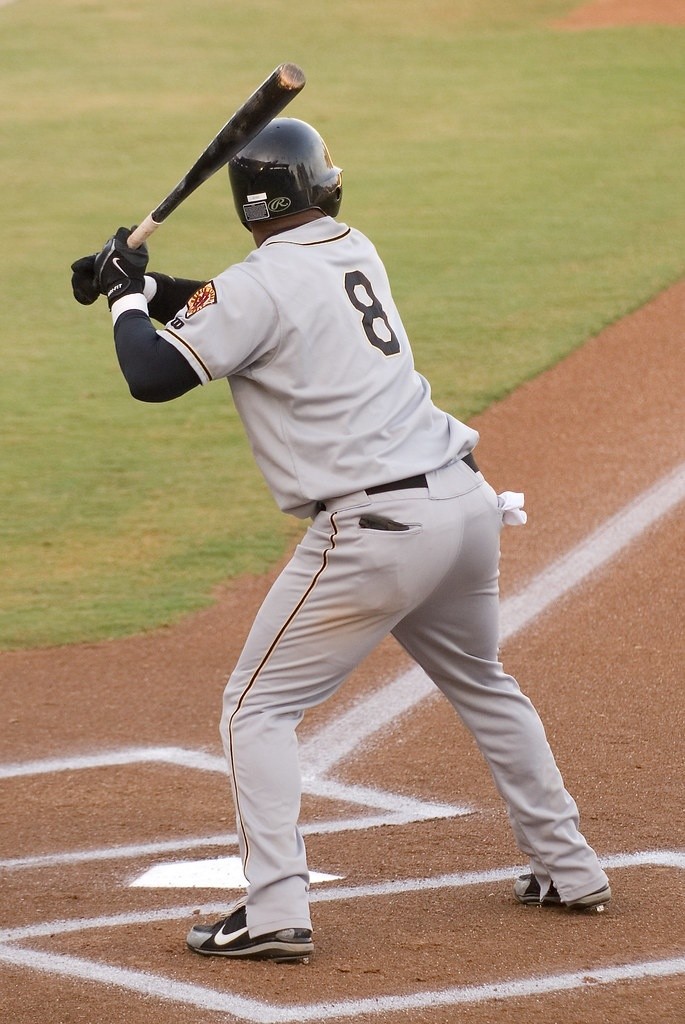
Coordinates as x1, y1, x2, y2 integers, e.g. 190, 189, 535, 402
229, 118, 342, 232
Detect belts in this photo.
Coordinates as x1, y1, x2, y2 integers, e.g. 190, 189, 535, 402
320, 451, 480, 512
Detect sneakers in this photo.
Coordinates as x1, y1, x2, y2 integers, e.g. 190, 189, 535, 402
186, 906, 314, 961
513, 873, 612, 907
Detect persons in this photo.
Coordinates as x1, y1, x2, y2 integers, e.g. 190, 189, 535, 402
71, 117, 614, 959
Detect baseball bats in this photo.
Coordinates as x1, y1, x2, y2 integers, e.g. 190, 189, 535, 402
127, 63, 307, 249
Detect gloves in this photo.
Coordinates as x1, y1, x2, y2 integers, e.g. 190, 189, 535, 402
72, 225, 149, 310
